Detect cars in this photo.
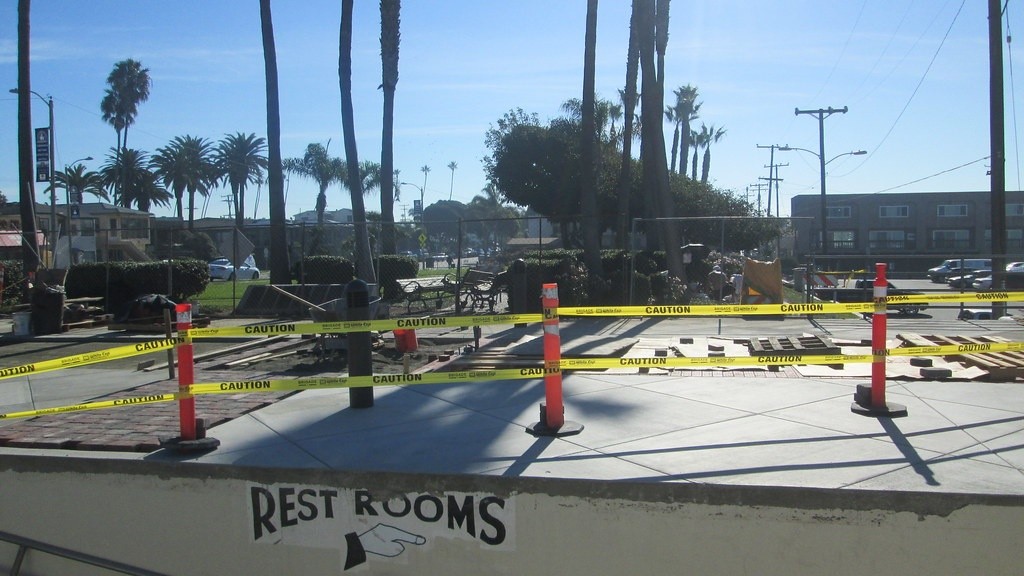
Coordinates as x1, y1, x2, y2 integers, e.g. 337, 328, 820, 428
943, 261, 1024, 292
206, 258, 262, 282
399, 245, 494, 261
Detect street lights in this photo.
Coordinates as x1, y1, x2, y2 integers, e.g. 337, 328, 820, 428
64, 156, 94, 265
9, 87, 58, 270
400, 182, 426, 270
778, 145, 867, 270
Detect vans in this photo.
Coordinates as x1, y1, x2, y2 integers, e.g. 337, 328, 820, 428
926, 258, 992, 285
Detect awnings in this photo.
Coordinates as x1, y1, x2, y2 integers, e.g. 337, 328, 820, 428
0, 230, 50, 246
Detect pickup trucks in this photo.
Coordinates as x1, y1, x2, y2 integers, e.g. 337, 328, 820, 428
814, 279, 929, 319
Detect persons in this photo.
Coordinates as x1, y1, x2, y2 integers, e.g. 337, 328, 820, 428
447, 255, 454, 269
708, 266, 728, 298
730, 269, 742, 302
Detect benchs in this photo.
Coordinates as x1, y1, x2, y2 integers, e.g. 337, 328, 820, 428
400, 267, 509, 315
